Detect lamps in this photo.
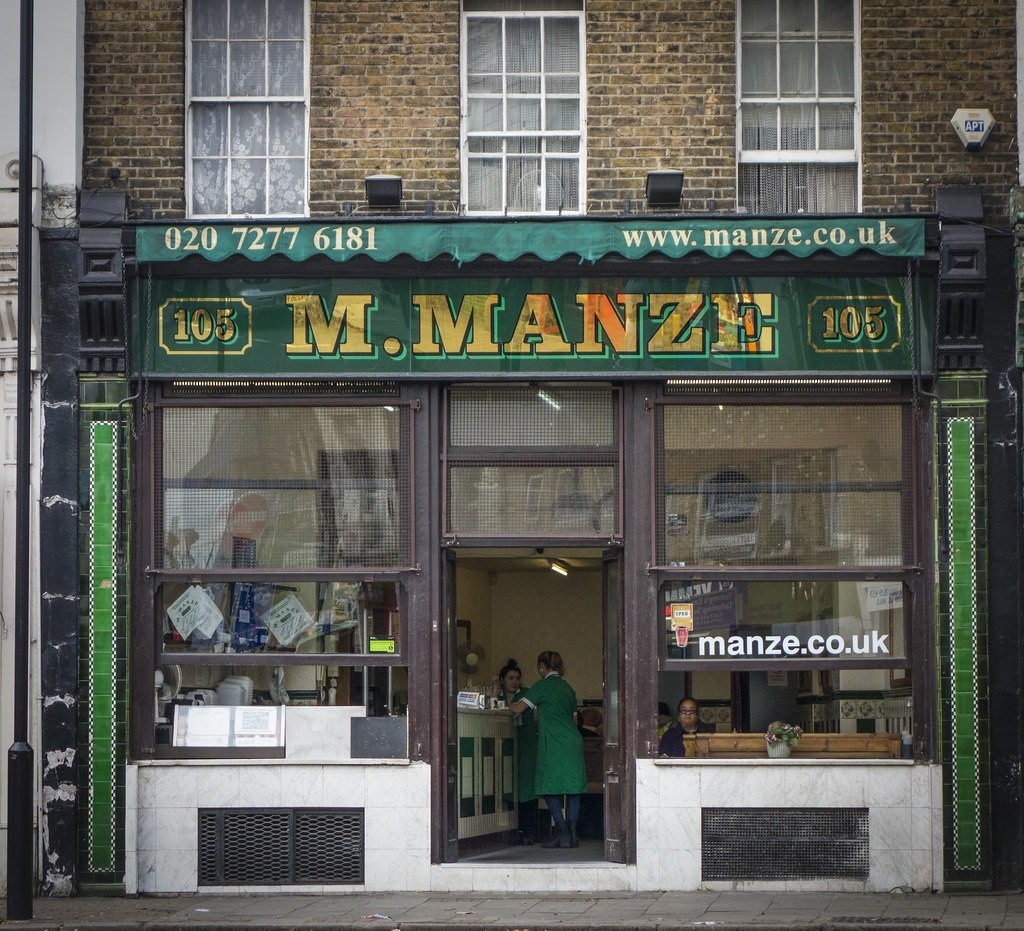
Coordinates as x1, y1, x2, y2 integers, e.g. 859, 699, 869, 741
547, 563, 569, 577
364, 175, 405, 208
643, 168, 685, 208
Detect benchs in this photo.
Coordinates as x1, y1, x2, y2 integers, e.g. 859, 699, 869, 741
682, 733, 902, 760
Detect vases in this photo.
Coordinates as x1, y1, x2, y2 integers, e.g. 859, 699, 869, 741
767, 739, 793, 757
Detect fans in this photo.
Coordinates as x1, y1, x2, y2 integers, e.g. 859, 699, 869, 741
153, 663, 183, 724
454, 637, 488, 674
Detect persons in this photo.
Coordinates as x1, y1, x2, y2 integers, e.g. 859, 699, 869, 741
509, 651, 586, 847
491, 658, 542, 845
659, 697, 713, 758
658, 701, 674, 744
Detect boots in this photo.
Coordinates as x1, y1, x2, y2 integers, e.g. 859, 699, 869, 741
541, 820, 578, 847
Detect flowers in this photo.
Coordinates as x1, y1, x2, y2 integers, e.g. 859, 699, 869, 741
765, 722, 805, 749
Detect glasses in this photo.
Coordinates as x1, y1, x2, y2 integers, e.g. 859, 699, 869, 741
679, 709, 697, 714
